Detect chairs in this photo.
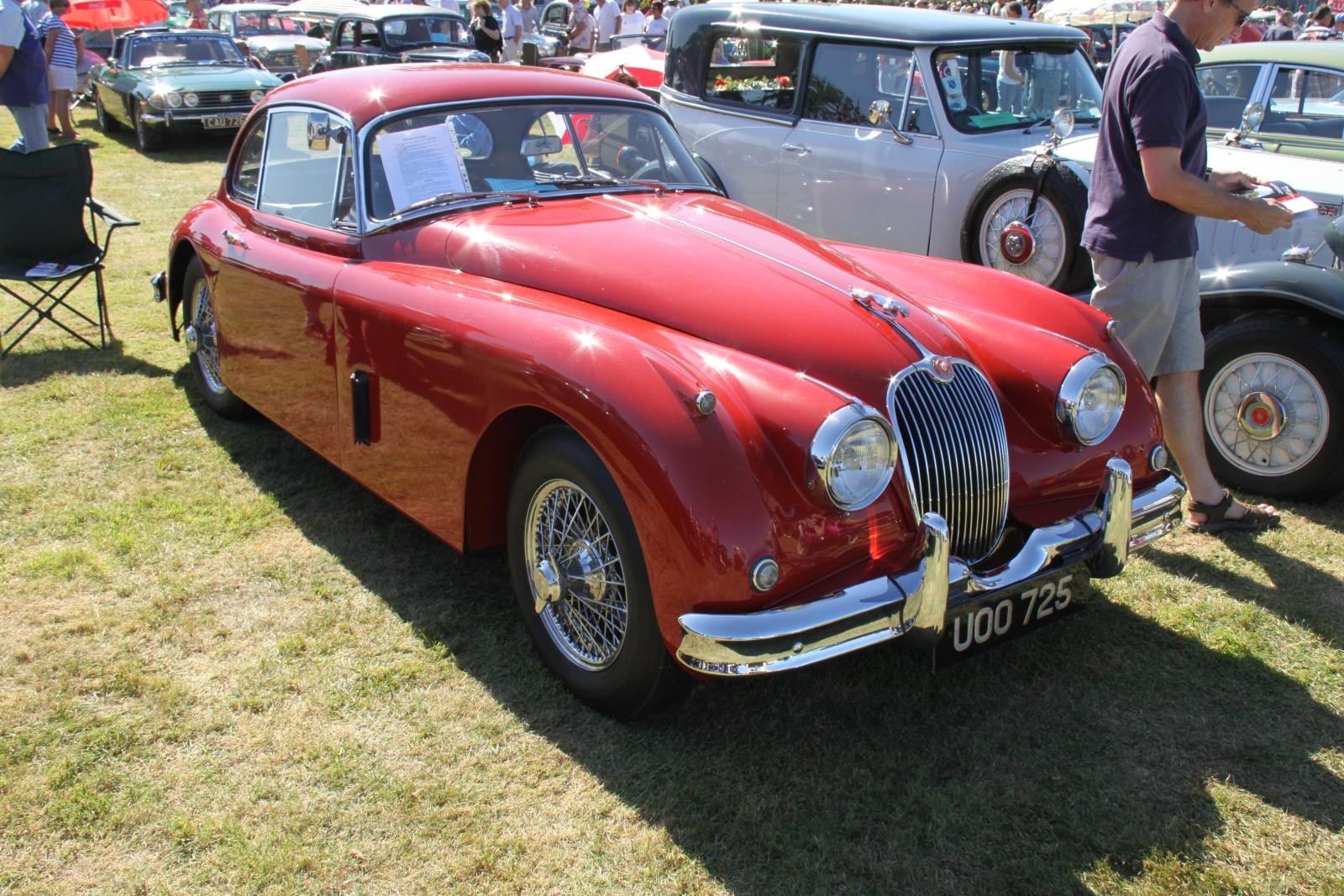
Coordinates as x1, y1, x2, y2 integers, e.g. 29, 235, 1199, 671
187, 45, 213, 60
1263, 117, 1344, 138
0, 143, 141, 359
131, 44, 156, 65
295, 45, 310, 77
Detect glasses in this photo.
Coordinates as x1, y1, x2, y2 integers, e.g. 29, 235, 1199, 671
1229, 0, 1250, 27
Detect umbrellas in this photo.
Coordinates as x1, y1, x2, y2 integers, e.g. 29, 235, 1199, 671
46, 0, 169, 38
544, 44, 667, 146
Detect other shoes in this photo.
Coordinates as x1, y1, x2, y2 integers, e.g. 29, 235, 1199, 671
63, 128, 81, 138
47, 127, 63, 136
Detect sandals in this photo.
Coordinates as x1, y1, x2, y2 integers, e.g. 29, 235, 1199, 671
1184, 489, 1281, 533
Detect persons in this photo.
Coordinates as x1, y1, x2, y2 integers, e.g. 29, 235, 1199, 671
579, 73, 640, 167
897, 0, 1063, 116
1230, 3, 1344, 99
377, 0, 729, 67
186, 0, 208, 29
0, 0, 103, 153
1079, 0, 1281, 531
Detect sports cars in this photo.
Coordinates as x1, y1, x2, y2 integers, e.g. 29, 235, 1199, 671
149, 60, 1194, 728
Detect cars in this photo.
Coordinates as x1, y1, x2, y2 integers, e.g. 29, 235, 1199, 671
580, 0, 1344, 511
176, 0, 574, 167
87, 28, 287, 155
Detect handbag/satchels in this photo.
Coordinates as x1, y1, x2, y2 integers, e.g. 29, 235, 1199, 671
36, 15, 48, 49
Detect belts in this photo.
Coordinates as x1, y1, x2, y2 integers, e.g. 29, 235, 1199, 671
504, 37, 513, 40
